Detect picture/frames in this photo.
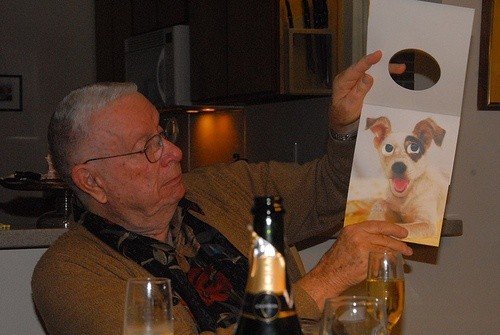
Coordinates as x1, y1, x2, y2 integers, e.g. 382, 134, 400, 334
0, 74, 23, 112
476, 0, 500, 111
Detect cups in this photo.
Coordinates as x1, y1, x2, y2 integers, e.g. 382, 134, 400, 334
322, 294, 389, 335
122, 277, 174, 335
363, 250, 403, 335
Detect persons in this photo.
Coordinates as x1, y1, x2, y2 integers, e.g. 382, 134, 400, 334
31, 50, 413, 335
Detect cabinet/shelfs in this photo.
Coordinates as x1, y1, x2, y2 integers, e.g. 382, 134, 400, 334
94, 0, 345, 112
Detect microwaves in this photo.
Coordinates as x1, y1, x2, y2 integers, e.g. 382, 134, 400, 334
123, 25, 192, 108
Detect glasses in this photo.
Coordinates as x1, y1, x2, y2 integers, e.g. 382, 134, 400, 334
84, 116, 181, 164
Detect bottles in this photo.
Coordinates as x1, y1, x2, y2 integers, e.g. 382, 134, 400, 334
233, 195, 303, 335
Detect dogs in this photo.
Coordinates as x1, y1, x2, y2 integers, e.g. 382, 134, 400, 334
362, 116, 448, 239
